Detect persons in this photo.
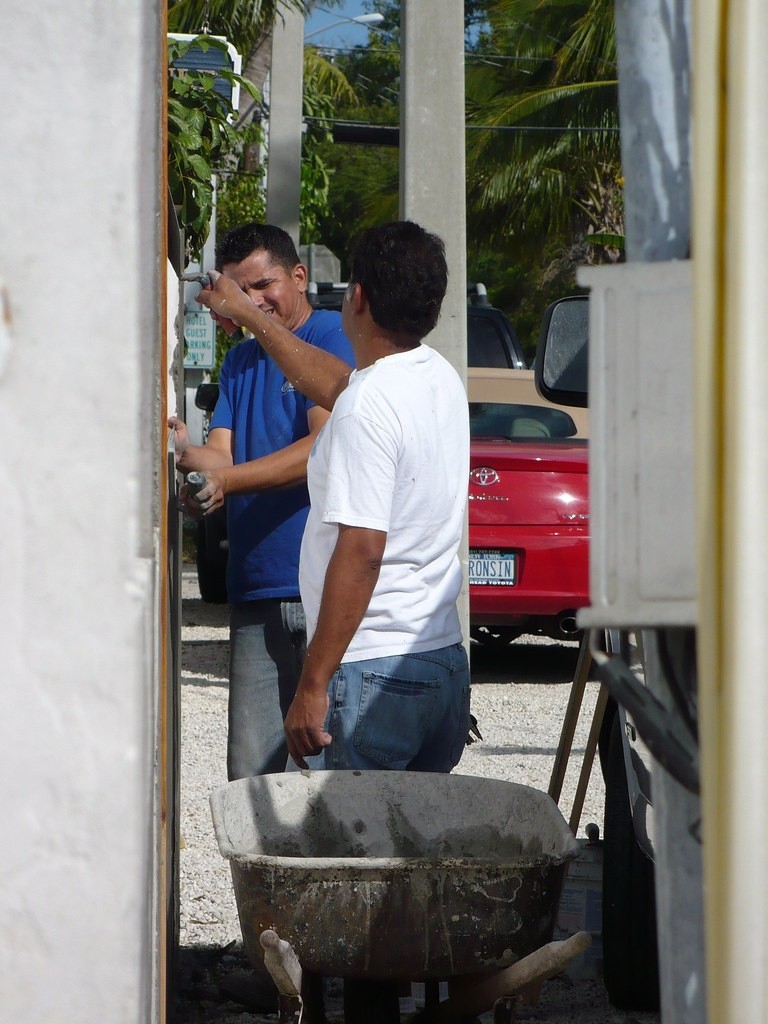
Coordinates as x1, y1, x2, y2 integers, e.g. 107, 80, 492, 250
168, 221, 357, 783
194, 216, 471, 774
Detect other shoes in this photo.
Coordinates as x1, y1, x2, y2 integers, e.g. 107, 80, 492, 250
222, 973, 277, 1012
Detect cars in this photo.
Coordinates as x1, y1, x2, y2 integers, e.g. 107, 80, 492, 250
468, 363, 588, 647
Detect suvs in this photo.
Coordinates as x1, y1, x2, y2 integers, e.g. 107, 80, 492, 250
309, 278, 524, 369
